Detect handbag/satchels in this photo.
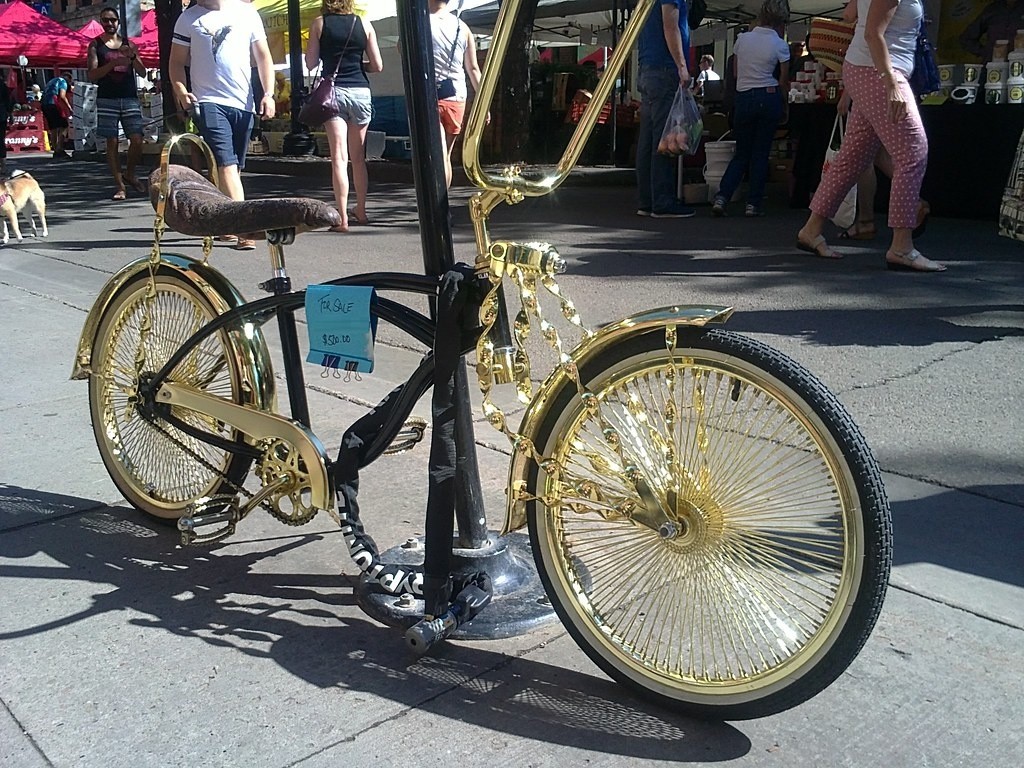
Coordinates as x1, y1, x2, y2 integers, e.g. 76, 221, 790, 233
658, 83, 704, 157
822, 111, 859, 228
809, 14, 861, 73
298, 79, 340, 128
909, 0, 942, 96
436, 79, 456, 100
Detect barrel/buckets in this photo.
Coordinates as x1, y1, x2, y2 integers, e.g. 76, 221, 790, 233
704, 141, 737, 171
703, 169, 745, 204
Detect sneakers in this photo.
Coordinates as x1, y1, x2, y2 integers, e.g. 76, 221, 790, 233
638, 200, 650, 216
650, 203, 697, 217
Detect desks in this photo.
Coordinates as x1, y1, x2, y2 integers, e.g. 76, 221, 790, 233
786, 102, 1024, 212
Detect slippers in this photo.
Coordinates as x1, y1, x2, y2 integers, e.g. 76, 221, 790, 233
350, 206, 368, 224
327, 226, 350, 232
112, 190, 127, 200
123, 174, 146, 193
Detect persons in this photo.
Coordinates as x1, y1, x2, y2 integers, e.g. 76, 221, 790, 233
149, 77, 160, 93
396, 0, 491, 226
304, 0, 383, 232
31, 71, 77, 163
959, 0, 1024, 91
0, 80, 16, 175
86, 8, 147, 200
168, 0, 276, 249
272, 72, 291, 118
636, 0, 949, 274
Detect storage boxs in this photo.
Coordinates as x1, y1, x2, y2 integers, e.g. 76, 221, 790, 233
383, 136, 412, 160
72, 81, 163, 154
170, 144, 191, 155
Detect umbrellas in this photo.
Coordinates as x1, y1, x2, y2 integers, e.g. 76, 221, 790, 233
248, 0, 367, 92
461, 0, 939, 162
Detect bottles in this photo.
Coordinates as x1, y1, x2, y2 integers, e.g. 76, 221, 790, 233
114, 38, 129, 72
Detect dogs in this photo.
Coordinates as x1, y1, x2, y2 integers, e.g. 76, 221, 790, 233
0, 171, 48, 243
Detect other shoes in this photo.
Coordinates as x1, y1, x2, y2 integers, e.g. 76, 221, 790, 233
745, 203, 766, 216
710, 199, 729, 216
219, 232, 240, 243
236, 234, 256, 249
53, 152, 71, 162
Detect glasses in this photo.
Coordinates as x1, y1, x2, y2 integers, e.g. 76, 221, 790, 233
101, 17, 118, 24
698, 60, 708, 65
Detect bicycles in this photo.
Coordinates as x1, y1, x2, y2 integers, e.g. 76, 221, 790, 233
70, 0, 896, 722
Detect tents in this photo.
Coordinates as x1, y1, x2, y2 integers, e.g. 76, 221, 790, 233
0, 0, 159, 85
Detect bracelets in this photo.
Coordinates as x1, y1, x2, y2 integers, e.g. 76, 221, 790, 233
131, 53, 137, 60
264, 93, 275, 100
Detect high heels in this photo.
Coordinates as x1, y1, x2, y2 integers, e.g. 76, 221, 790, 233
837, 219, 875, 240
912, 197, 930, 239
886, 248, 946, 272
796, 234, 845, 259
